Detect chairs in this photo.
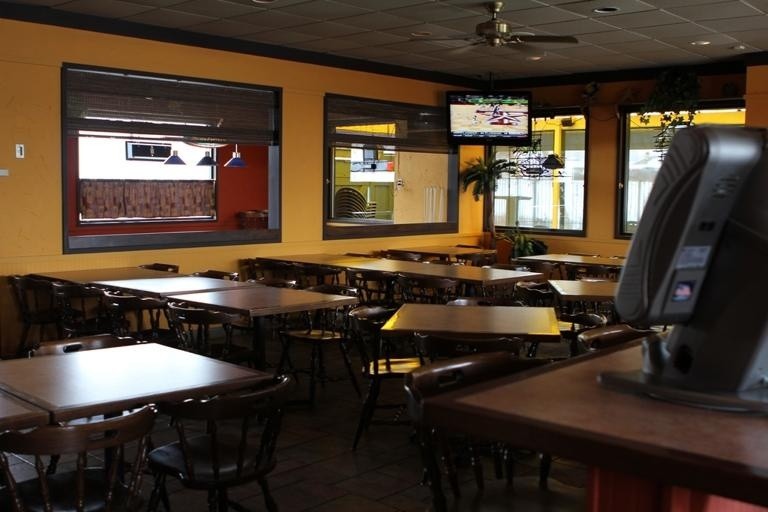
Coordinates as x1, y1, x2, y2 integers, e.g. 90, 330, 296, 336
0, 244, 656, 512
335, 187, 377, 219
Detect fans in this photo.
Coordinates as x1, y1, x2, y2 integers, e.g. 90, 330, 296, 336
409, 2, 579, 61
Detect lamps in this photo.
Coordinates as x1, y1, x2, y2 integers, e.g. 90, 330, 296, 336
224, 144, 247, 167
163, 150, 186, 164
196, 151, 218, 165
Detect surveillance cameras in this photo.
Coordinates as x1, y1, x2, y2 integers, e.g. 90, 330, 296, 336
581, 80, 601, 98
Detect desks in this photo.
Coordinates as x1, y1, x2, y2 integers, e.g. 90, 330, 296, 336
420, 329, 768, 512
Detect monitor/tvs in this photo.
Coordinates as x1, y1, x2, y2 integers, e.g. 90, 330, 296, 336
597, 124, 767, 413
446, 91, 532, 146
